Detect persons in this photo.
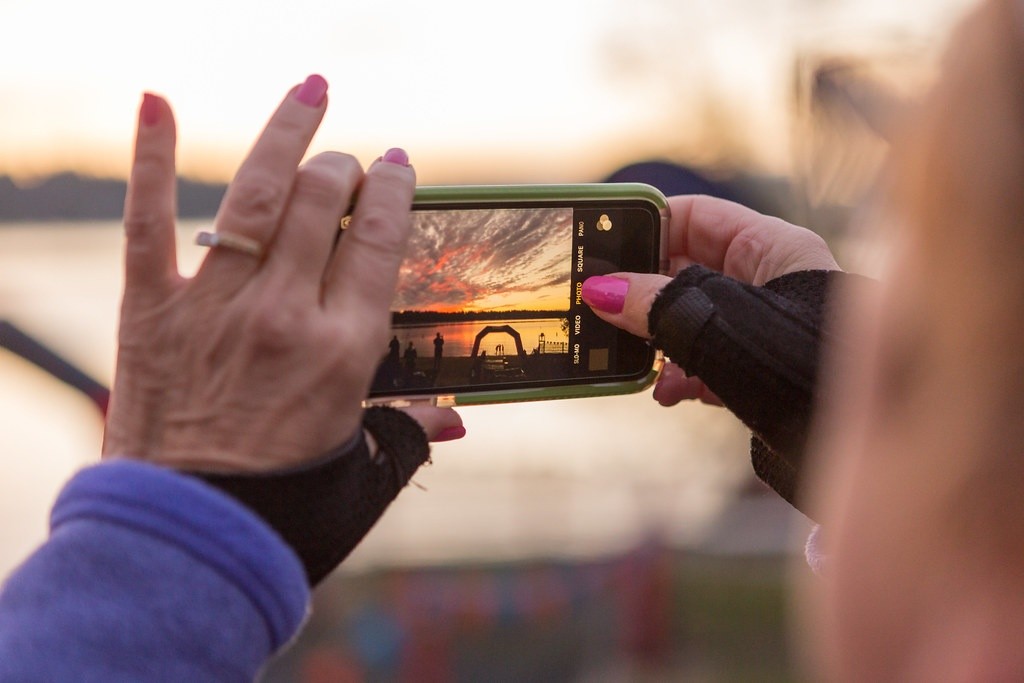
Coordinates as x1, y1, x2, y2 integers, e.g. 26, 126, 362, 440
1, 1, 1023, 682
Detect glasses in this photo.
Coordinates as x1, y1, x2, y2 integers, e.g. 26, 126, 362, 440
792, 50, 1024, 256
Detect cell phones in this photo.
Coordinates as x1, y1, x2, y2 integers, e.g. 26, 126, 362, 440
334, 183, 671, 410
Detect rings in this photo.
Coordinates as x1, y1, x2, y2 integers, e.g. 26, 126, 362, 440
197, 231, 268, 261
